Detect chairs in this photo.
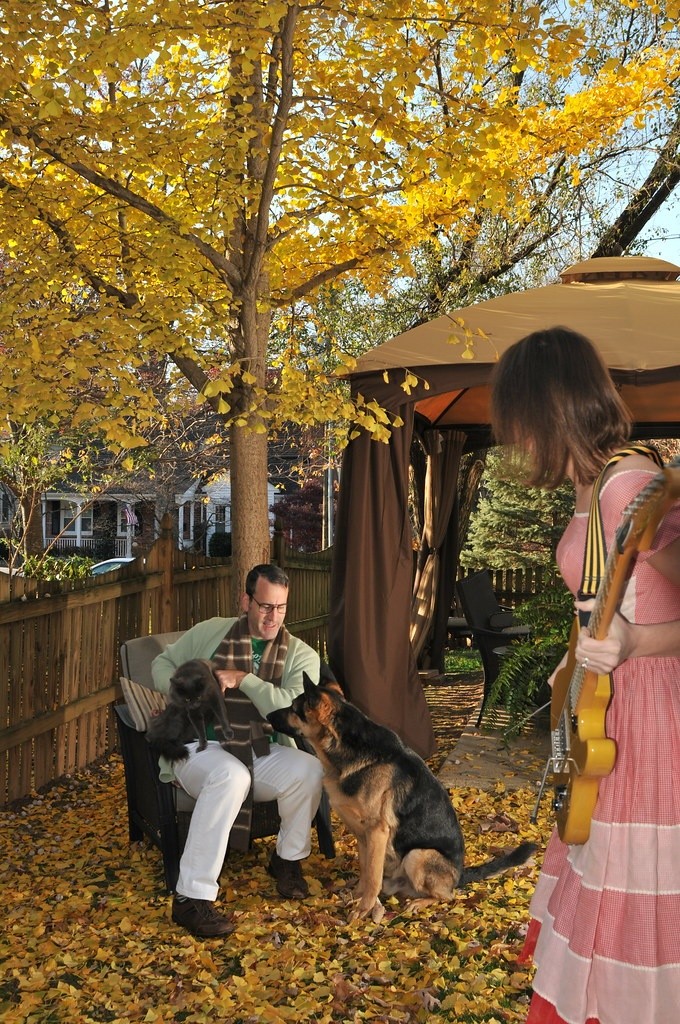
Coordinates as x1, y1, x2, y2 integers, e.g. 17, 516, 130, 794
456, 568, 549, 728
112, 630, 336, 896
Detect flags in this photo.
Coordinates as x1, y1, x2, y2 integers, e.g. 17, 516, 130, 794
123, 509, 139, 526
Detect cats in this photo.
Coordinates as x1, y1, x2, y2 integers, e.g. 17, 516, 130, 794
146, 657, 234, 764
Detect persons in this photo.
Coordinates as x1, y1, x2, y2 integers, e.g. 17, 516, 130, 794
153, 564, 323, 941
489, 326, 680, 1024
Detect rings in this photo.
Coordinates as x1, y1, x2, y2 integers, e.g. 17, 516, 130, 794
584, 658, 588, 663
581, 664, 585, 668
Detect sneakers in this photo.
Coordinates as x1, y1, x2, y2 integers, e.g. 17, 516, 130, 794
266, 851, 310, 899
171, 895, 233, 940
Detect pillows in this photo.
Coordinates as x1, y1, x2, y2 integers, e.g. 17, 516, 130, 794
120, 676, 168, 733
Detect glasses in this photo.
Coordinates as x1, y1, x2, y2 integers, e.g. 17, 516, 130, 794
249, 595, 288, 615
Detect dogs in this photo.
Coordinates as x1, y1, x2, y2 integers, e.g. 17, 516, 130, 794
265, 659, 538, 926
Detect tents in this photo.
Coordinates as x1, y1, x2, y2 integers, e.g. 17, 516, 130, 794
325, 255, 680, 761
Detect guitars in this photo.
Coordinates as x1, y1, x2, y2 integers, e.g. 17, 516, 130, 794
546, 463, 680, 847
